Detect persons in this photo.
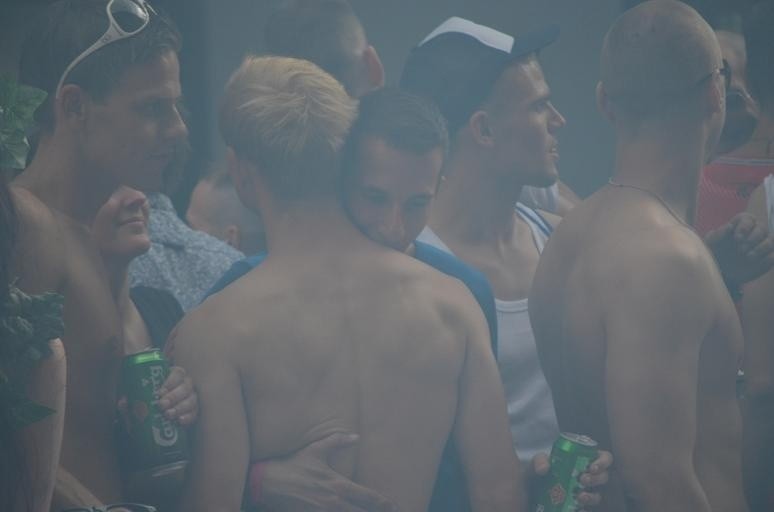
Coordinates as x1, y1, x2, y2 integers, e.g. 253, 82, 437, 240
0, 0, 774, 512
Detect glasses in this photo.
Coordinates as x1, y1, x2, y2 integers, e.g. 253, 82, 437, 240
697, 59, 731, 88
54, 0, 157, 101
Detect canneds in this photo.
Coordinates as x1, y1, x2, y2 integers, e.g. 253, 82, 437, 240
534, 432, 598, 512
121, 348, 188, 478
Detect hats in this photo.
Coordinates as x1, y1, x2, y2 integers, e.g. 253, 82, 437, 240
402, 16, 561, 131
